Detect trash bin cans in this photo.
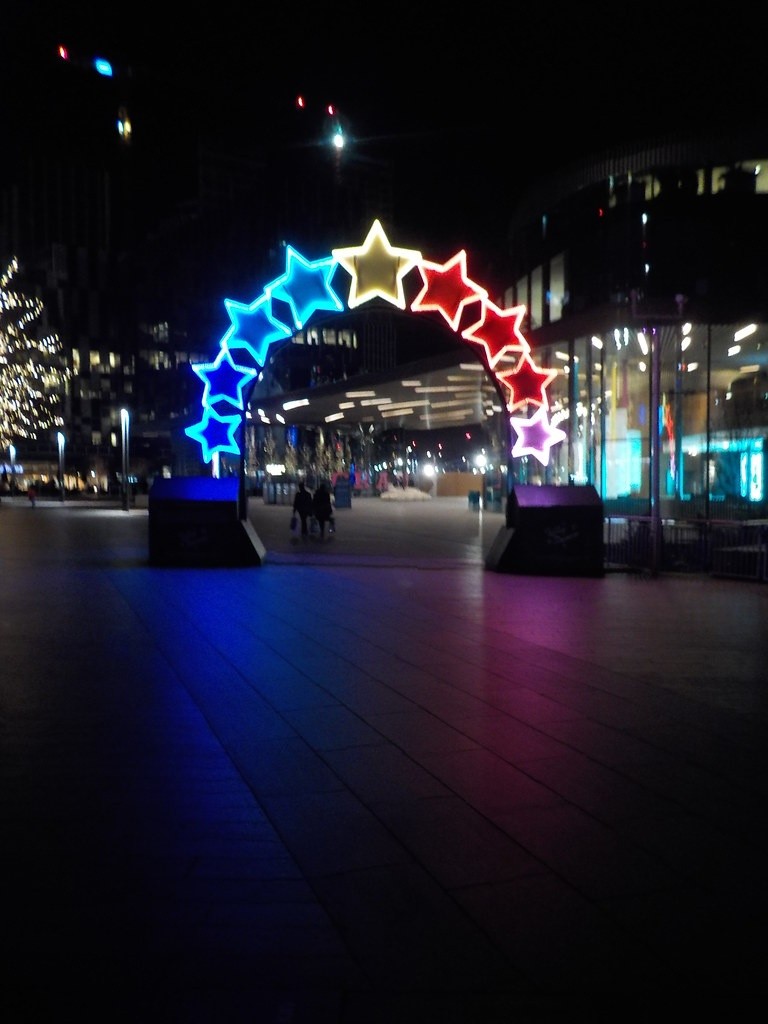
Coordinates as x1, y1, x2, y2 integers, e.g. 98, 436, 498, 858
485, 486, 502, 513
334, 482, 352, 509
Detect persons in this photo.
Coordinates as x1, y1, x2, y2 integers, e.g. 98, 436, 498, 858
336, 464, 410, 498
293, 482, 332, 541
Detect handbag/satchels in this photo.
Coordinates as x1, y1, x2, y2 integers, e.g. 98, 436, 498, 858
309, 518, 320, 533
290, 513, 297, 530
328, 518, 336, 533
